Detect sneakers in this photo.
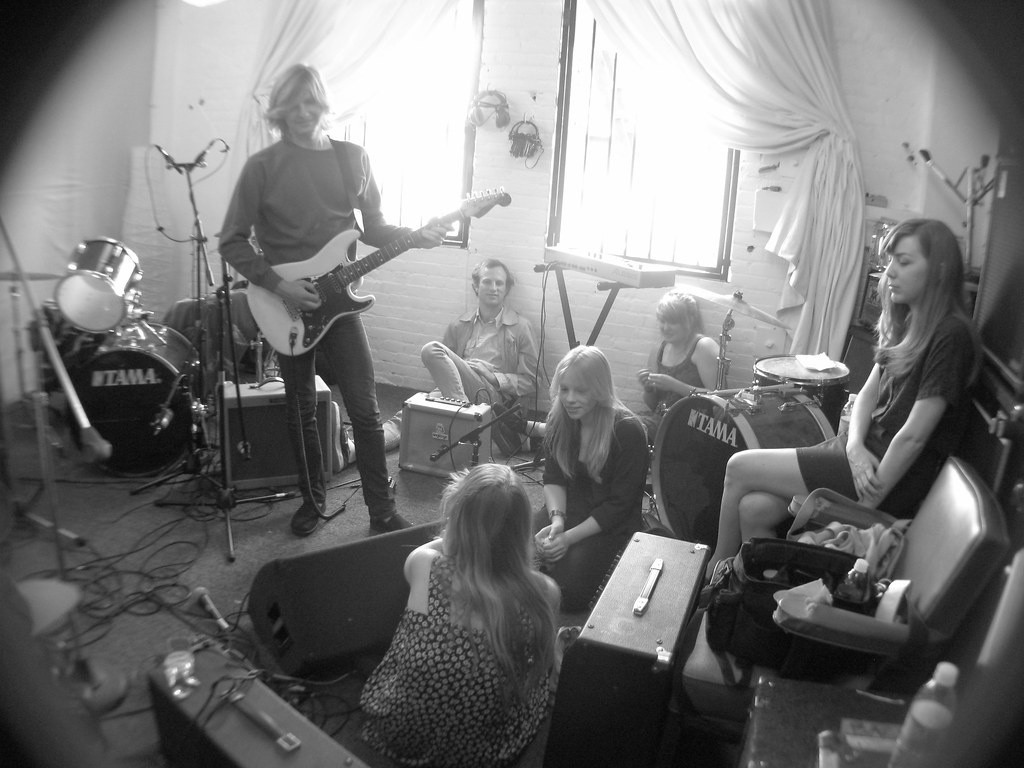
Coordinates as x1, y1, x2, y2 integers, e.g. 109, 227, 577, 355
291, 500, 326, 534
493, 402, 527, 434
491, 419, 522, 457
370, 513, 413, 532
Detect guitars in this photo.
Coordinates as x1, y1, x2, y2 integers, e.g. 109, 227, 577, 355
247, 186, 512, 357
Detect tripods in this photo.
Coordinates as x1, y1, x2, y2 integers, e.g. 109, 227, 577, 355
154, 252, 294, 562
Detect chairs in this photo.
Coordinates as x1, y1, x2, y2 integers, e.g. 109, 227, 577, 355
682, 455, 1010, 742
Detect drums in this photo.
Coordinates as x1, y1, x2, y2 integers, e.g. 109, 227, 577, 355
61, 321, 199, 478
54, 236, 143, 335
650, 386, 837, 562
752, 353, 850, 436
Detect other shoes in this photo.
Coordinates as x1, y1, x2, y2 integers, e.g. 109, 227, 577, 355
331, 401, 350, 471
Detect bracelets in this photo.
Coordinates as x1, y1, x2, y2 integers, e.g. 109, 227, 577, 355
550, 510, 568, 522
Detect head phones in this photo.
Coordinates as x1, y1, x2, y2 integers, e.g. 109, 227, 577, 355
509, 121, 540, 159
469, 91, 510, 128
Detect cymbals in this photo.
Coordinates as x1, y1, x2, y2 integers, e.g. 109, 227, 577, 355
675, 284, 791, 330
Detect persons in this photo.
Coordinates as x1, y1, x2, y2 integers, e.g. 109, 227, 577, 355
490, 289, 721, 458
218, 65, 453, 536
332, 257, 542, 471
358, 463, 561, 768
701, 218, 982, 599
526, 346, 648, 613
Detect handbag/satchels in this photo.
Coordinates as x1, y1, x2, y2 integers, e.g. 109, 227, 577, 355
696, 538, 878, 692
797, 519, 913, 594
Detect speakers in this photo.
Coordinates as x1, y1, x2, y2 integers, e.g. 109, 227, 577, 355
399, 393, 492, 477
542, 536, 716, 768
247, 518, 447, 676
220, 379, 332, 491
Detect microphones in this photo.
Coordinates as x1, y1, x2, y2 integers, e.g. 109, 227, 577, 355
155, 407, 175, 435
156, 145, 183, 175
236, 440, 251, 460
430, 445, 449, 461
194, 586, 229, 632
189, 141, 214, 172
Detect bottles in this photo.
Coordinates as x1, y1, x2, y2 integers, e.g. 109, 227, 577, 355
833, 558, 869, 602
885, 660, 960, 768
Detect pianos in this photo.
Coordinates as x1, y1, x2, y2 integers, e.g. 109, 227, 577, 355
530, 243, 678, 472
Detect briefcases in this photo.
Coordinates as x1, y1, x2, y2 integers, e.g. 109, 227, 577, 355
573, 532, 709, 708
220, 374, 333, 490
146, 646, 367, 768
